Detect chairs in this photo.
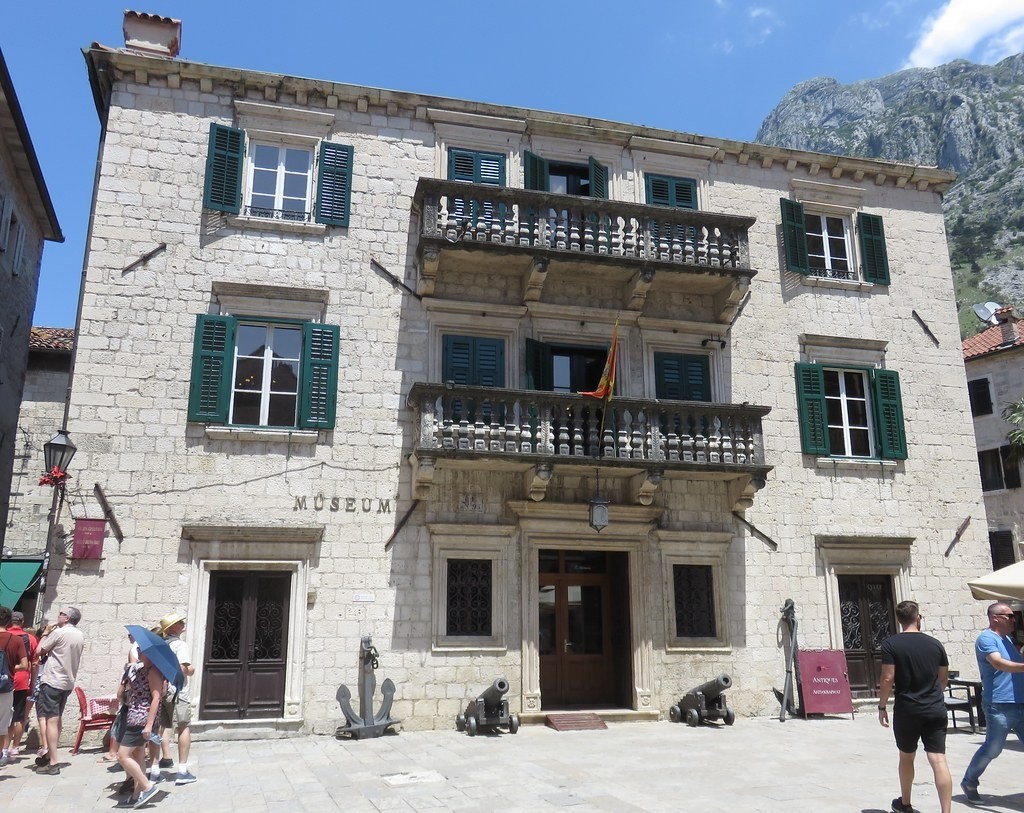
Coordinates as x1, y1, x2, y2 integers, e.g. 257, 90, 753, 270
72, 687, 114, 755
943, 688, 976, 736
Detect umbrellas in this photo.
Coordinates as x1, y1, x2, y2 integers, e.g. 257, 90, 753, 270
966, 560, 1024, 601
121, 625, 184, 691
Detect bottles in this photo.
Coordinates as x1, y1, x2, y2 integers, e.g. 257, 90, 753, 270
141, 730, 164, 745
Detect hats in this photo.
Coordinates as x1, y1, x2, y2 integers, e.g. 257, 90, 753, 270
12, 611, 25, 621
157, 612, 187, 635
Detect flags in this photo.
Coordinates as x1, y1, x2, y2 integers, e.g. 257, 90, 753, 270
577, 318, 618, 402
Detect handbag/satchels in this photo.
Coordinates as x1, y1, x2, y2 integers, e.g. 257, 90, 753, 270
110, 703, 129, 743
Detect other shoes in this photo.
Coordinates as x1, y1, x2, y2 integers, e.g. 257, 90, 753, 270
36, 761, 60, 775
148, 772, 167, 783
119, 777, 135, 794
107, 763, 125, 771
134, 784, 160, 809
35, 753, 50, 767
1, 746, 19, 757
116, 795, 138, 807
159, 757, 174, 768
174, 770, 197, 783
0, 756, 8, 766
960, 781, 986, 805
36, 747, 49, 757
891, 797, 914, 813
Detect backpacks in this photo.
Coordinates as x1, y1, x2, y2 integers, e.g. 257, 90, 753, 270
0, 633, 14, 694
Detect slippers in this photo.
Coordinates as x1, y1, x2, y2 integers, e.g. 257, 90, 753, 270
97, 754, 118, 763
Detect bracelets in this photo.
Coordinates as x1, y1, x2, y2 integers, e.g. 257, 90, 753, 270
878, 706, 886, 711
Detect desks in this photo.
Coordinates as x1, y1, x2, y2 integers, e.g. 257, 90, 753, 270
89, 698, 122, 719
949, 679, 986, 733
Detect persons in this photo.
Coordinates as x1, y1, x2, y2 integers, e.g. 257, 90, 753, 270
959, 603, 1024, 804
878, 600, 952, 813
96, 611, 197, 809
0, 606, 84, 775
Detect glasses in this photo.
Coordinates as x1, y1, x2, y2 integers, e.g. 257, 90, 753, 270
137, 647, 140, 652
128, 634, 131, 638
917, 614, 922, 619
995, 613, 1017, 620
60, 612, 67, 617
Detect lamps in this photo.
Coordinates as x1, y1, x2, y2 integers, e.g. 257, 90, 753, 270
43, 429, 77, 509
585, 468, 610, 533
